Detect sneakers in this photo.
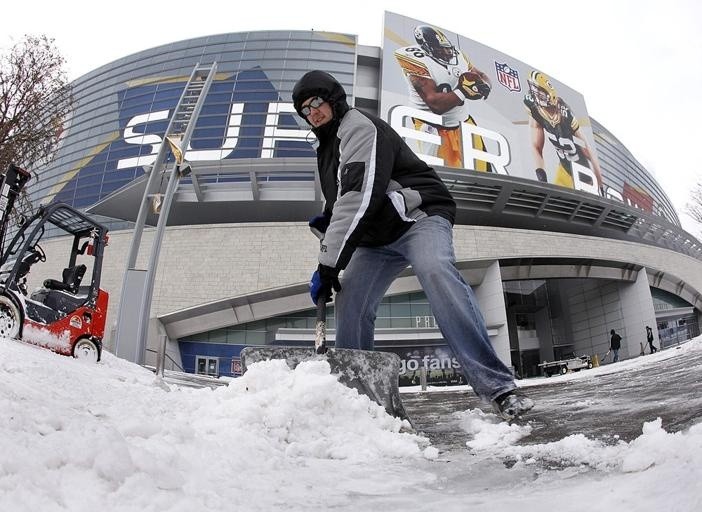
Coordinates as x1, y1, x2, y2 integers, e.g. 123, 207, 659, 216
492, 385, 535, 425
649, 348, 657, 354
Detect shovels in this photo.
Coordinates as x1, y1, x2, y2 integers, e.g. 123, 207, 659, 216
601, 350, 611, 361
239, 227, 418, 435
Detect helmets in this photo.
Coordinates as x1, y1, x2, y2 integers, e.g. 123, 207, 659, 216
412, 25, 460, 67
526, 69, 560, 112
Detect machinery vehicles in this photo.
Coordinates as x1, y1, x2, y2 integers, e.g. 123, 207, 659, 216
0, 160, 109, 364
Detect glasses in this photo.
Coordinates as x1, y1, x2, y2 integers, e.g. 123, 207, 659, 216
297, 94, 325, 118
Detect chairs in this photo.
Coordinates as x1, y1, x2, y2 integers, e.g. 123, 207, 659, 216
43, 264, 87, 294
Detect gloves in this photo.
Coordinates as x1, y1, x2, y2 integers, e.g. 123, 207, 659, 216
309, 261, 342, 307
535, 166, 547, 183
454, 71, 490, 104
307, 210, 332, 241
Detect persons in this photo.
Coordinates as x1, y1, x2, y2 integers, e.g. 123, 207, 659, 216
645, 325, 657, 354
291, 70, 535, 421
609, 328, 622, 362
522, 71, 605, 199
393, 25, 499, 174
